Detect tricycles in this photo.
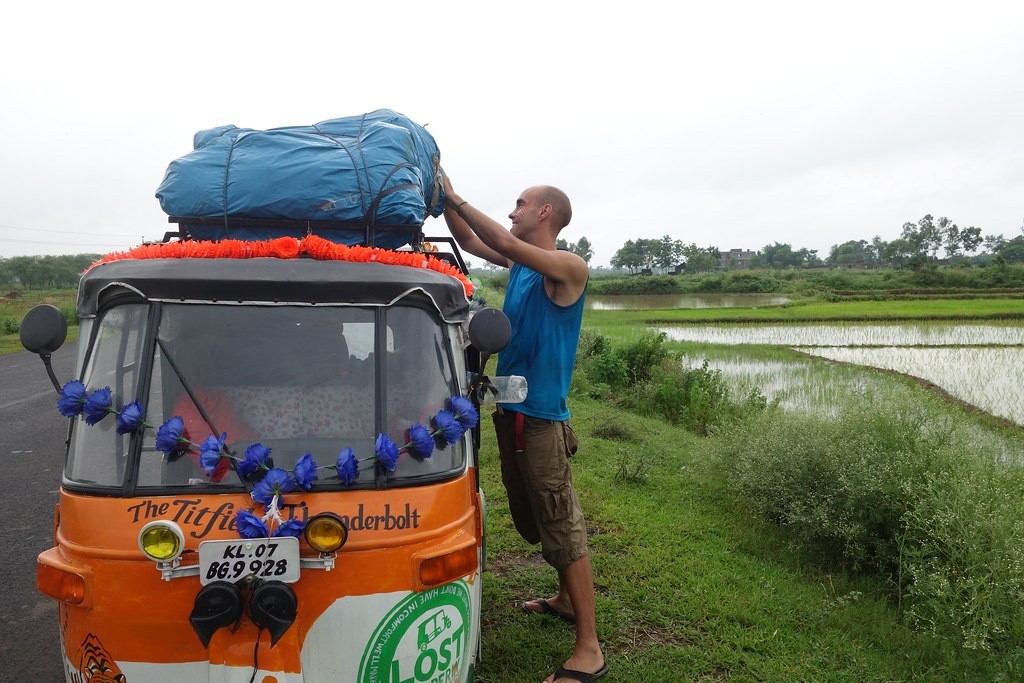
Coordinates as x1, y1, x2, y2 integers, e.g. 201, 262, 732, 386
16, 234, 514, 683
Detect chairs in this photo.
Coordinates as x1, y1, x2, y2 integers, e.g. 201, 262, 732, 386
166, 348, 422, 483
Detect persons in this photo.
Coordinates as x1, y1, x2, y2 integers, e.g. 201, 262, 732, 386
429, 153, 609, 683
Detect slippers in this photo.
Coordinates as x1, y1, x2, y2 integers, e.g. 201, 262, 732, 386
552, 662, 610, 682
522, 597, 575, 621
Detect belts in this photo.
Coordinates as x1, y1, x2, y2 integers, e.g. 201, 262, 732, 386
514, 412, 525, 453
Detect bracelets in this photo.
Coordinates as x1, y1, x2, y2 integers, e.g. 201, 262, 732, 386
442, 207, 455, 218
455, 200, 468, 213
